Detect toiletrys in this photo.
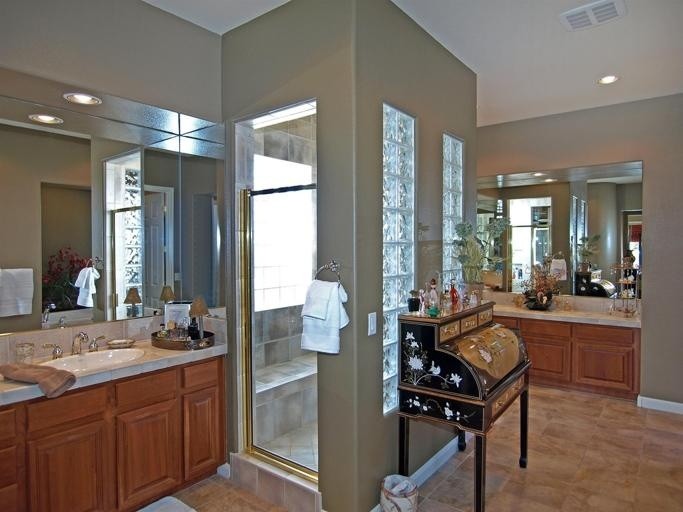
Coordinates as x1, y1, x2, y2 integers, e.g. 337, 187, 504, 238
155, 320, 187, 339
407, 279, 478, 318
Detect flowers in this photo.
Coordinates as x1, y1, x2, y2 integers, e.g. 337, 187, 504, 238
42, 245, 92, 310
511, 262, 567, 308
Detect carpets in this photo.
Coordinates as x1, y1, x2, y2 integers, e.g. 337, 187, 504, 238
138, 494, 197, 512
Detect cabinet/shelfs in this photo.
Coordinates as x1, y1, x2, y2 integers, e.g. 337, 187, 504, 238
26, 367, 180, 511
519, 320, 572, 382
0, 408, 19, 512
493, 316, 519, 330
575, 269, 617, 298
574, 326, 633, 391
183, 356, 222, 482
607, 264, 640, 317
397, 299, 533, 512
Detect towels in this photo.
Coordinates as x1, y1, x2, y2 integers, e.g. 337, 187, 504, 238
0, 362, 76, 398
74, 267, 100, 307
301, 280, 352, 354
0, 268, 35, 317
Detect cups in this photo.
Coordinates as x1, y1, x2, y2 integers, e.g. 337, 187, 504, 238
16, 343, 34, 363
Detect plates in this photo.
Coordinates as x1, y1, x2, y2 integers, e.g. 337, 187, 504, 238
106, 338, 135, 348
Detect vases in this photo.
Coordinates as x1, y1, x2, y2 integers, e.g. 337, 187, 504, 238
526, 296, 552, 311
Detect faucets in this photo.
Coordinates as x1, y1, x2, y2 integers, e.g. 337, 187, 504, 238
70, 331, 89, 355
42, 303, 57, 323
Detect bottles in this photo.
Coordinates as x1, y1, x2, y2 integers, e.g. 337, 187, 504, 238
408, 277, 480, 319
157, 316, 199, 341
511, 265, 522, 279
624, 250, 635, 264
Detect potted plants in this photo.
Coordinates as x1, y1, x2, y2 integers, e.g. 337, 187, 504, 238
477, 217, 511, 291
451, 220, 485, 299
622, 249, 635, 267
578, 233, 600, 272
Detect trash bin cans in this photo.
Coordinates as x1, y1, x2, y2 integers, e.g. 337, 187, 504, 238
379, 474, 418, 511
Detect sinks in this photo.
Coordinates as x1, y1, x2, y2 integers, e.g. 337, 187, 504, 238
37, 348, 145, 375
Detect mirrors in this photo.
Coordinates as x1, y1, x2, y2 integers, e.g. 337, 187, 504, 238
477, 160, 643, 299
0, 95, 226, 333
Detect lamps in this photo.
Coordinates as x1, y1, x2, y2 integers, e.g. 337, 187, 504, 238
160, 286, 176, 303
189, 296, 211, 338
123, 288, 142, 317
